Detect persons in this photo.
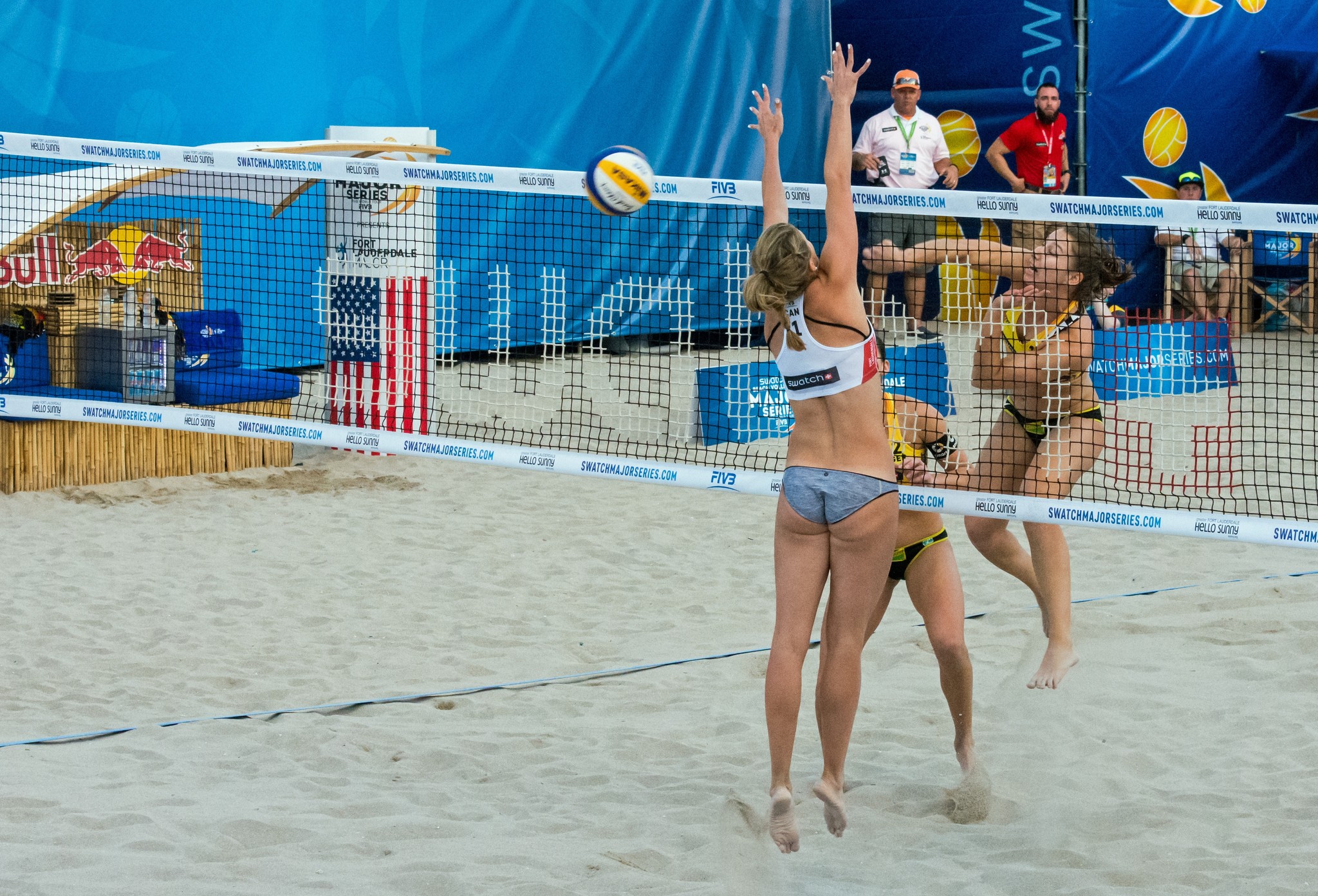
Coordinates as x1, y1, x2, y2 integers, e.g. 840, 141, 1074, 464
864, 222, 1141, 692
815, 335, 993, 825
854, 68, 959, 341
986, 83, 1071, 291
747, 41, 898, 855
1154, 172, 1244, 338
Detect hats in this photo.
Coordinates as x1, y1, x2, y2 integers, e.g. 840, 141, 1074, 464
1176, 171, 1204, 189
893, 69, 920, 90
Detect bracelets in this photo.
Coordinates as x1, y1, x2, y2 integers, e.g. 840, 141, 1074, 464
927, 429, 959, 459
949, 163, 959, 173
1060, 170, 1072, 177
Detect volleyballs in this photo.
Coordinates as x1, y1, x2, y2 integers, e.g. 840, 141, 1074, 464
584, 144, 655, 216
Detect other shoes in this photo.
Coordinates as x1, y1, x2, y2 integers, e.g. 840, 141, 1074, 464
906, 326, 942, 342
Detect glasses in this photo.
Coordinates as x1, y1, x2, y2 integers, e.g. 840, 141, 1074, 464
1181, 177, 1200, 182
893, 77, 920, 85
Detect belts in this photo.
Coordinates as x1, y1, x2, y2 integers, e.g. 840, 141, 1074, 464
1025, 183, 1061, 195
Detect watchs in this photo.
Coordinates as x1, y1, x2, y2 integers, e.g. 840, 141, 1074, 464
1182, 234, 1190, 244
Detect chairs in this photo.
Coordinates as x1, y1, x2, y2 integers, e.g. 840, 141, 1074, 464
170, 309, 300, 406
1164, 230, 1318, 338
0, 333, 125, 424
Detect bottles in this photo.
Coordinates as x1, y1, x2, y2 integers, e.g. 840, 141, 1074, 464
143, 288, 156, 328
123, 287, 137, 328
98, 289, 111, 327
